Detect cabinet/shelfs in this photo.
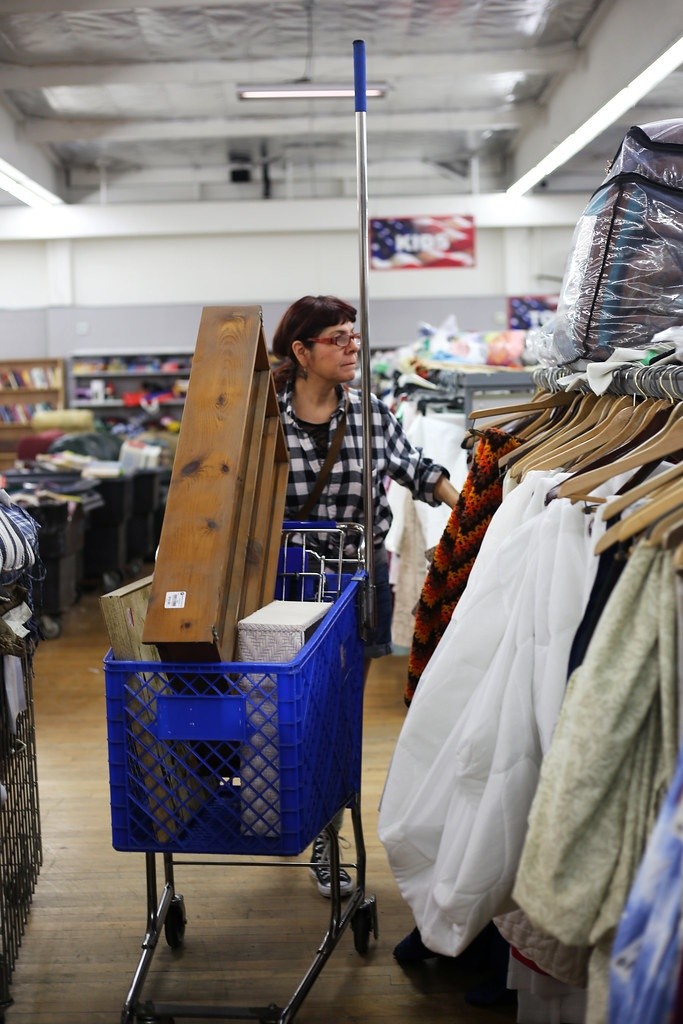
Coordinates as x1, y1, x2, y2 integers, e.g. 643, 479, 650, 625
0, 351, 191, 464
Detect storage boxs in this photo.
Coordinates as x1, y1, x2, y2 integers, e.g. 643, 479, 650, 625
13, 464, 175, 624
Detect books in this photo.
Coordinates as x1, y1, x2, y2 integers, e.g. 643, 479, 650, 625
36, 449, 121, 478
1, 365, 61, 425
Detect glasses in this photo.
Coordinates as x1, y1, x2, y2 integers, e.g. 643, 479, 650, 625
302, 332, 363, 348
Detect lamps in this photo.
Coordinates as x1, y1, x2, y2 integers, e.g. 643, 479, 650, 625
238, 79, 391, 100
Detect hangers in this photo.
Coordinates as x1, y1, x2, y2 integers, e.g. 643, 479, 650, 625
389, 354, 682, 576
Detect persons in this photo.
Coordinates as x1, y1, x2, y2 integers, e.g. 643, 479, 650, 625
271, 297, 463, 899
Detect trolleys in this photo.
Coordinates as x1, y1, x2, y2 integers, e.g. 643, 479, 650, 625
104, 521, 371, 1024
